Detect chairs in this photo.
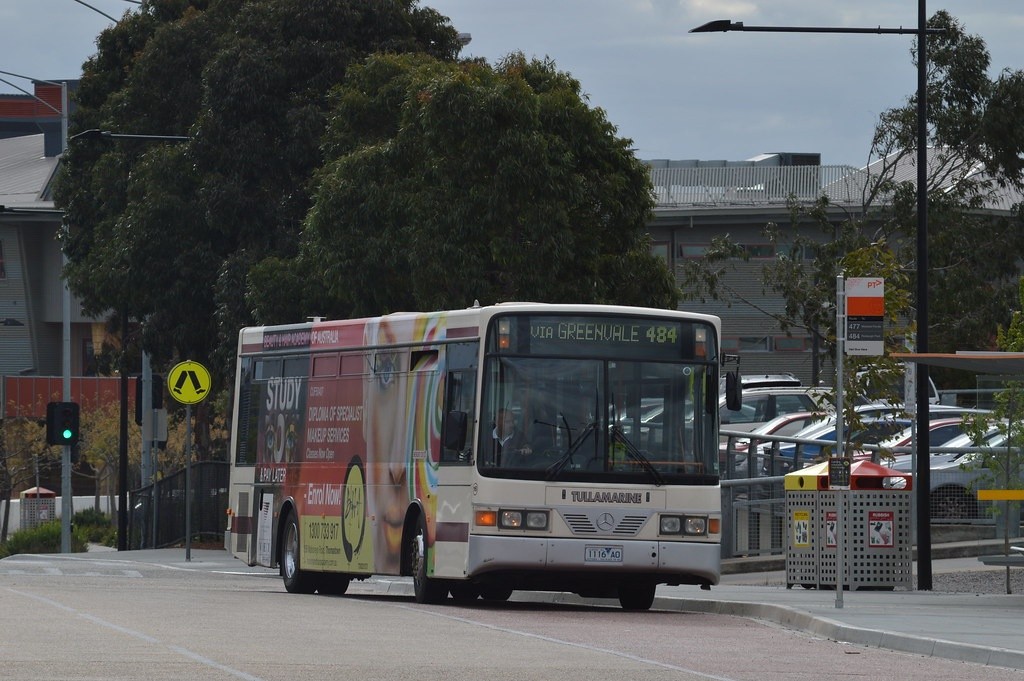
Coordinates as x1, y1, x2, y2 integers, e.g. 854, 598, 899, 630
445, 410, 596, 468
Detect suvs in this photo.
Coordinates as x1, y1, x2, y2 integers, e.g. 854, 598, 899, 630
720, 372, 803, 411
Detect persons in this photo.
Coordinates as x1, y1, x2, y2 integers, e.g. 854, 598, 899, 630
489, 407, 533, 466
361, 316, 410, 574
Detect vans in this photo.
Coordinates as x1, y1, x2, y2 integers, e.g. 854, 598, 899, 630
856, 360, 941, 414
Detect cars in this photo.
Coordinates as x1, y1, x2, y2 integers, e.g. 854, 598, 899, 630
876, 420, 1024, 524
716, 403, 1009, 468
607, 401, 693, 443
684, 390, 836, 443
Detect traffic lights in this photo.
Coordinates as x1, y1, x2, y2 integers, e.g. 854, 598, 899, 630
47, 402, 80, 446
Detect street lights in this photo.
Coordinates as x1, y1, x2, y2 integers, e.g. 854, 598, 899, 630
689, 21, 933, 590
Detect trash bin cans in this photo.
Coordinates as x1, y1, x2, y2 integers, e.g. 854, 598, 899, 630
19, 486, 56, 532
784, 460, 915, 592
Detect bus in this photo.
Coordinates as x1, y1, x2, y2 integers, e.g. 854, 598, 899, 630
223, 300, 742, 610
